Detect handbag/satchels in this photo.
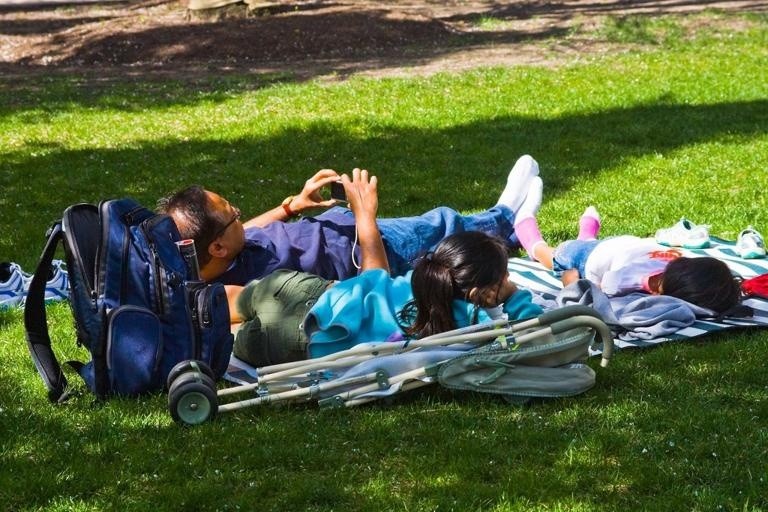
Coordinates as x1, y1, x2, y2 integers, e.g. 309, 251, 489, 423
60, 196, 235, 398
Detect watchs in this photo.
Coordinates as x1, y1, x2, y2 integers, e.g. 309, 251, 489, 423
280, 195, 299, 219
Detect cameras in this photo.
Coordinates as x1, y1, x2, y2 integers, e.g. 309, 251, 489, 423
330, 181, 348, 204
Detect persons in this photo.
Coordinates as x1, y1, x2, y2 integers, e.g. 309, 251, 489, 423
153, 153, 546, 288
220, 229, 545, 369
512, 205, 744, 322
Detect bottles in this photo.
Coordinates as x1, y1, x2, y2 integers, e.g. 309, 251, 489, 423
176, 239, 201, 282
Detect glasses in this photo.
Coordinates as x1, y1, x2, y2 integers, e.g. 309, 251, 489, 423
212, 205, 242, 241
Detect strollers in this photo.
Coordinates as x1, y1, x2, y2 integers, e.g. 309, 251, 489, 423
166, 305, 615, 428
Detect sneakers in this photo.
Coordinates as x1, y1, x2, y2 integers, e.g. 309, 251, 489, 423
0, 258, 74, 309
654, 216, 710, 248
737, 228, 766, 259
